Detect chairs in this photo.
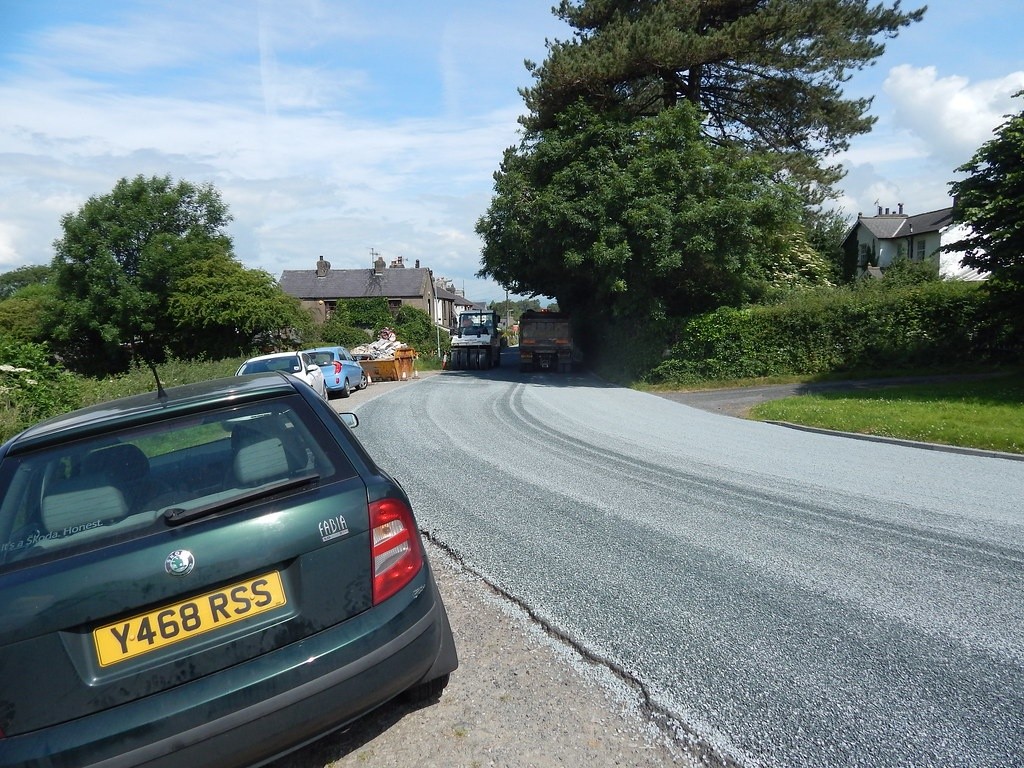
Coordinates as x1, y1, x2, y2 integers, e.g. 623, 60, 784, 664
254, 363, 270, 374
88, 444, 171, 508
289, 359, 299, 369
484, 320, 491, 326
226, 415, 284, 484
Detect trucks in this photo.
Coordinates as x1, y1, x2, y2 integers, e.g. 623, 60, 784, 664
518, 310, 576, 374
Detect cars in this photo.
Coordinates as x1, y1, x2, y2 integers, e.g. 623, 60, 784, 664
225, 350, 328, 429
0, 372, 462, 768
303, 346, 368, 398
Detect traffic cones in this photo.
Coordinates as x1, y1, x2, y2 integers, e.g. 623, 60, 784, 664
442, 352, 450, 371
366, 371, 373, 386
411, 368, 420, 379
400, 370, 409, 381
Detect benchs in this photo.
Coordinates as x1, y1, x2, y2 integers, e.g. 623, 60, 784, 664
40, 426, 307, 536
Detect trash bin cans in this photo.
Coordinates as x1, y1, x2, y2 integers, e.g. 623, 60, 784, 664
353, 348, 416, 382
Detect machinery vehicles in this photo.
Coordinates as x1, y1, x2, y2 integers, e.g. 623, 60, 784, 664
450, 310, 502, 371
